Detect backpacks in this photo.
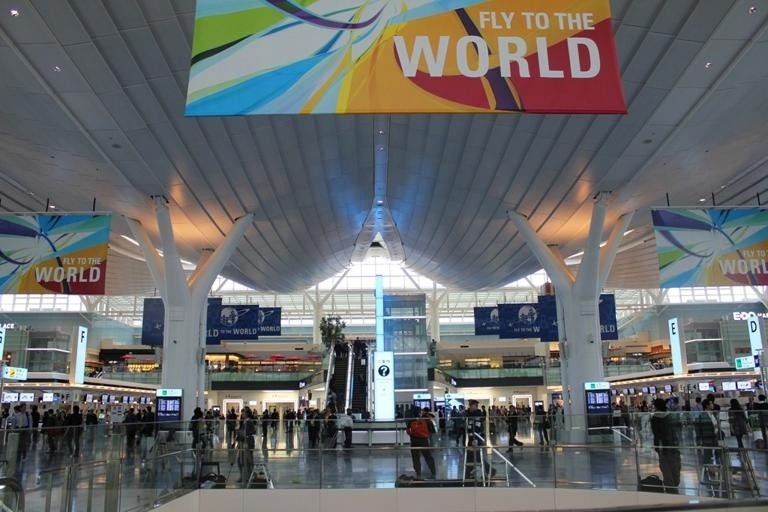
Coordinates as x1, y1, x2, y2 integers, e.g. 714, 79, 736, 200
409, 420, 428, 439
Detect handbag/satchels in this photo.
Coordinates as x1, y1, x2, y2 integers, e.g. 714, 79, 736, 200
637, 475, 663, 492
545, 421, 550, 428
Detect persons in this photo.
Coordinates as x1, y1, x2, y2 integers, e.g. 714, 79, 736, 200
461, 399, 497, 487
121, 406, 155, 457
334, 336, 369, 358
359, 407, 370, 419
613, 394, 768, 480
481, 403, 564, 453
430, 339, 437, 356
395, 403, 466, 447
649, 398, 682, 494
86, 409, 111, 442
327, 390, 346, 413
1, 402, 83, 472
261, 405, 356, 455
189, 406, 258, 480
406, 406, 436, 480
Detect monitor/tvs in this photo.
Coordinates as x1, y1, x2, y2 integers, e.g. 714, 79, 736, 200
722, 382, 737, 391
85, 393, 93, 403
100, 394, 150, 405
19, 392, 35, 402
737, 381, 751, 390
698, 383, 709, 391
664, 384, 673, 393
610, 384, 657, 396
42, 393, 54, 402
3, 393, 19, 403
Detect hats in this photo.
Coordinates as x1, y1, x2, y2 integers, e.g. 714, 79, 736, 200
468, 400, 479, 405
